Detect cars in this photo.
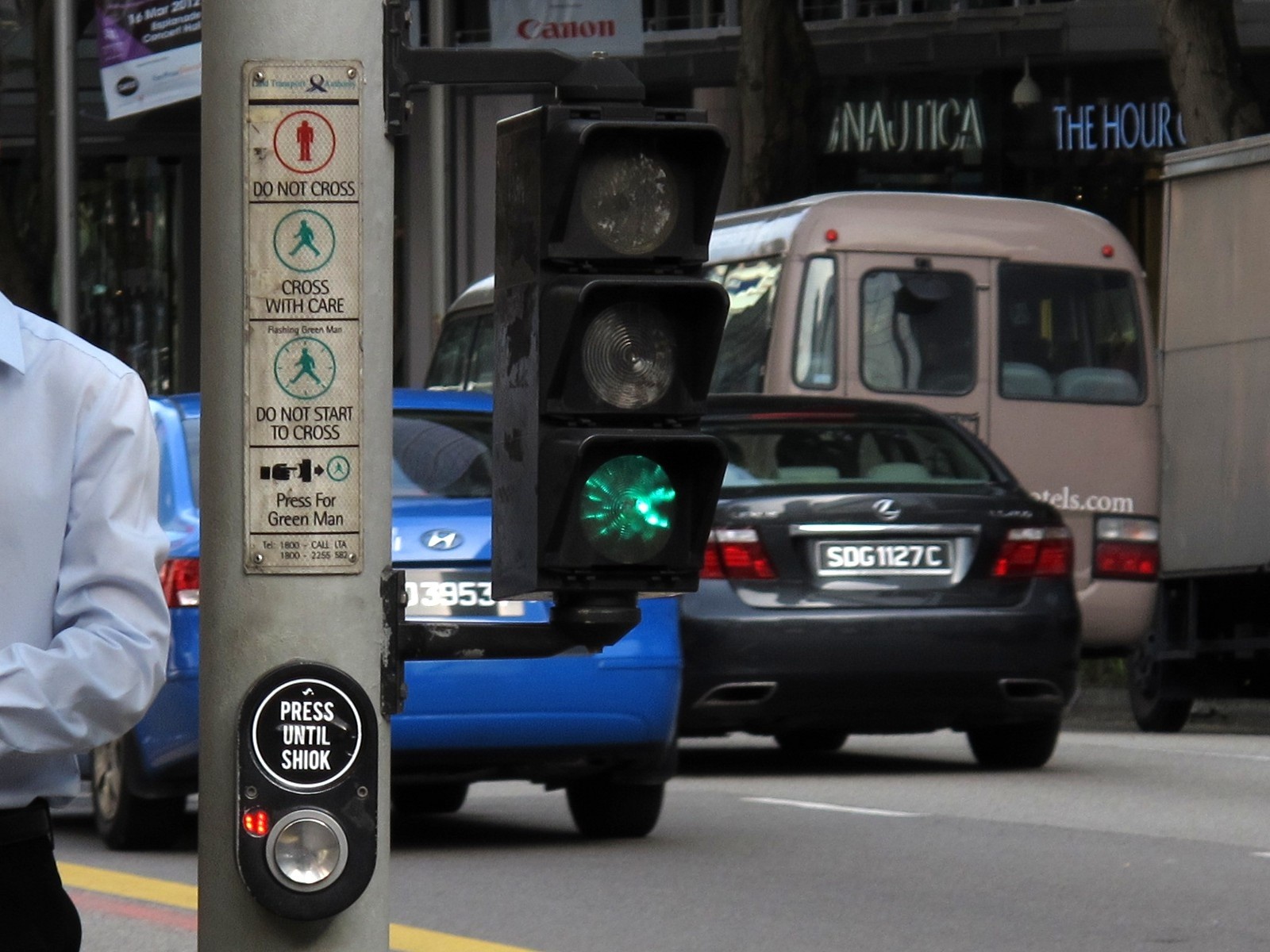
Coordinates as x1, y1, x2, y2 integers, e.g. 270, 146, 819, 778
674, 390, 1081, 770
79, 390, 685, 840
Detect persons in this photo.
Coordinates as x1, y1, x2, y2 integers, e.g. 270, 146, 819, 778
1, 282, 171, 952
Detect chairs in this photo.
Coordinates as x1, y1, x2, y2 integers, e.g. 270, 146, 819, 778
1003, 363, 1055, 402
1057, 365, 1139, 403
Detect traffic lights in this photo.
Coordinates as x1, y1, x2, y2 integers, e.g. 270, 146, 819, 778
489, 104, 731, 589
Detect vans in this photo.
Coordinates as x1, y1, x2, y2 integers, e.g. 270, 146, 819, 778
420, 191, 1160, 652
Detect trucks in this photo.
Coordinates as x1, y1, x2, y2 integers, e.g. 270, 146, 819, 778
1126, 135, 1270, 732
